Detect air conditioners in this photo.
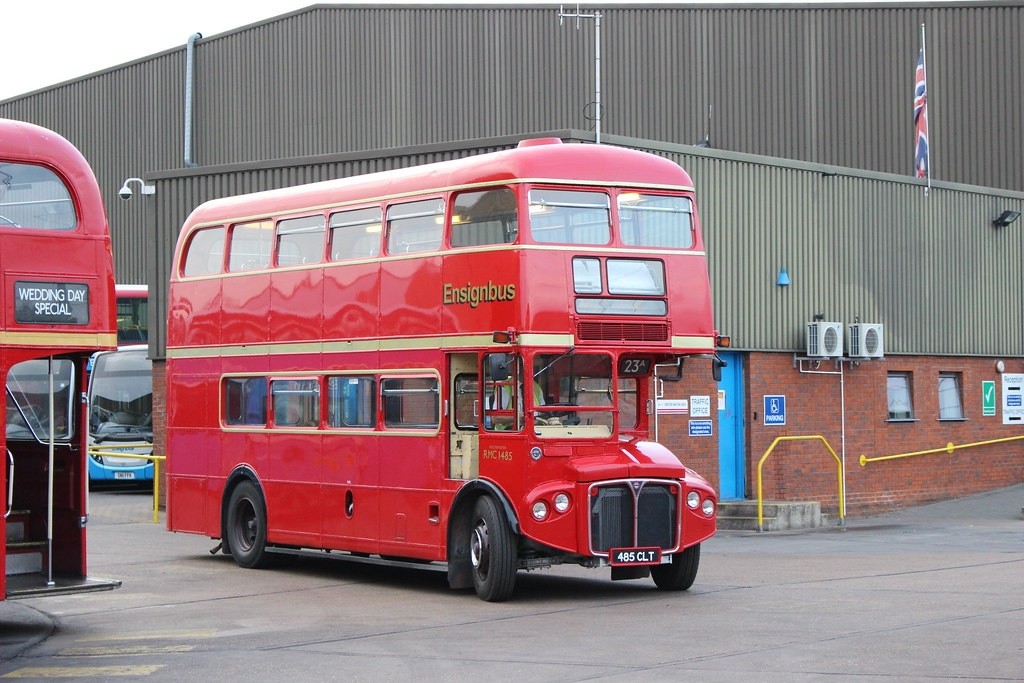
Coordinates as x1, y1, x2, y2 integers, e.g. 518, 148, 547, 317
806, 321, 844, 357
847, 323, 884, 358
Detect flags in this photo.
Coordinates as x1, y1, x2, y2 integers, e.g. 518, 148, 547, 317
914, 48, 929, 180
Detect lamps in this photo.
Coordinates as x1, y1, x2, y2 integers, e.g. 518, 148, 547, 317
992, 210, 1022, 226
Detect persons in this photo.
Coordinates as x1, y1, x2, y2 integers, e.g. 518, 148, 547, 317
492, 356, 545, 430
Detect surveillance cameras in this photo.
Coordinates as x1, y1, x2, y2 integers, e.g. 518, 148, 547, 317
118, 186, 133, 200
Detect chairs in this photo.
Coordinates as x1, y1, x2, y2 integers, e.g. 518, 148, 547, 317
241, 244, 424, 270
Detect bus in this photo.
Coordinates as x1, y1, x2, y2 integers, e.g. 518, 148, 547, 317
0, 117, 123, 603
166, 137, 731, 602
87, 284, 155, 489
